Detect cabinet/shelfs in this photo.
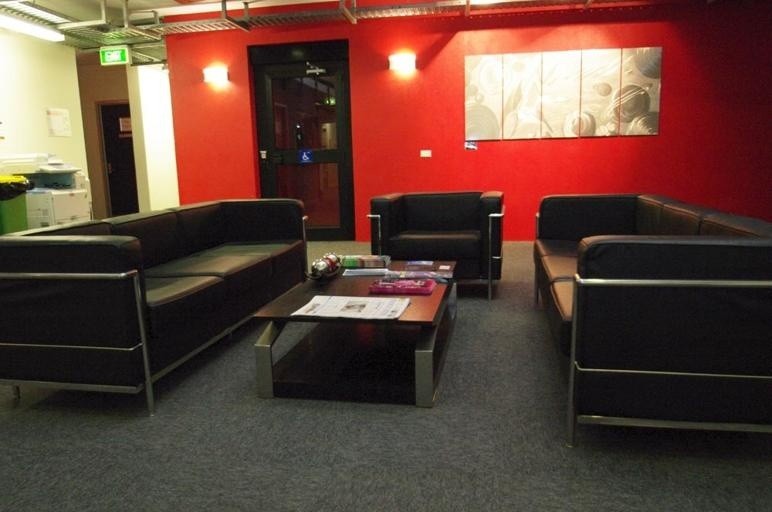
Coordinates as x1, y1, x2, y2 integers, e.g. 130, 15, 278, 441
24, 188, 89, 231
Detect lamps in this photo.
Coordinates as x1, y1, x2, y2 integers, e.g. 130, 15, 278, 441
386, 54, 420, 75
199, 65, 230, 86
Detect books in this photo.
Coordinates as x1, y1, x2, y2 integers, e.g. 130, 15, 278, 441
339, 251, 457, 296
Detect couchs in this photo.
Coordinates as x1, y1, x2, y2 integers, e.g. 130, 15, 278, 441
0, 197, 310, 420
532, 191, 772, 449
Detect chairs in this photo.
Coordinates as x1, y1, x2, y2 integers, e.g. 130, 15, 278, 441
365, 190, 506, 301
